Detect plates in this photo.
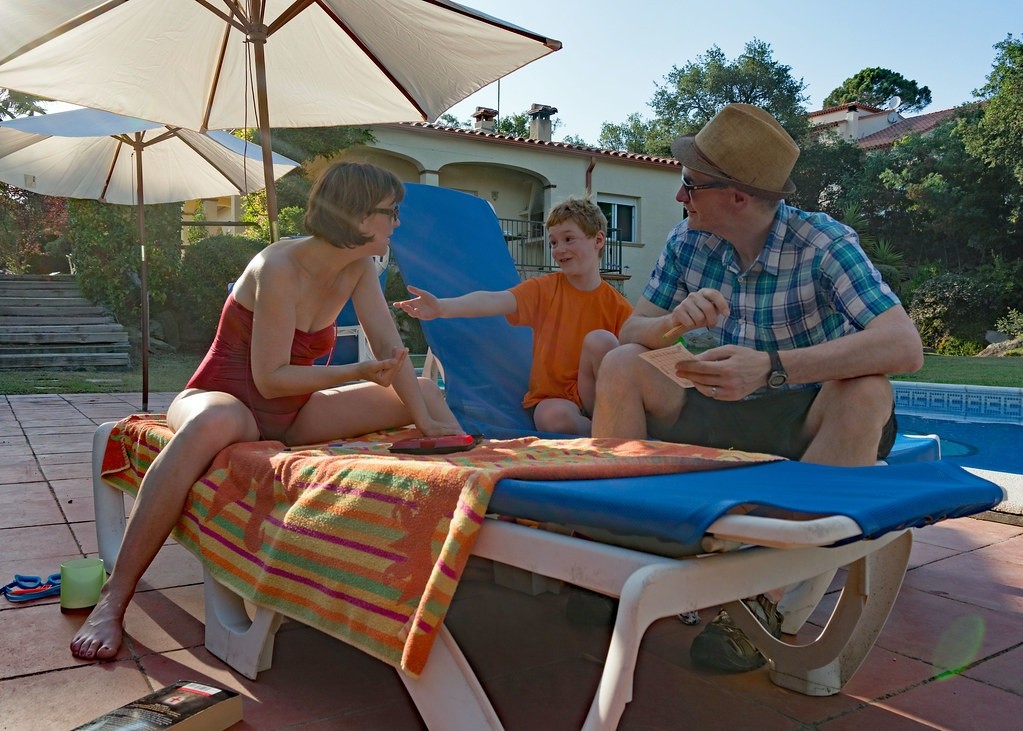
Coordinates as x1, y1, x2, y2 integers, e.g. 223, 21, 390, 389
387, 433, 484, 455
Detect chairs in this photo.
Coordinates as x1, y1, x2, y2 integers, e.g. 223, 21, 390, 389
389, 182, 941, 635
92, 416, 1004, 731
227, 236, 390, 385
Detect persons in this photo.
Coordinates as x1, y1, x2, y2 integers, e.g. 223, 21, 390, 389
392, 187, 636, 436
566, 102, 925, 672
70, 162, 468, 655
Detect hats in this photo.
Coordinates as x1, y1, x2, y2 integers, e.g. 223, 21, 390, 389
670, 103, 800, 199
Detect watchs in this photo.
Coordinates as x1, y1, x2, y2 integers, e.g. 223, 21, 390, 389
765, 347, 788, 391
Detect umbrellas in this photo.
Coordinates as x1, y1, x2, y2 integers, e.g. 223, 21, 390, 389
0, 107, 302, 410
0, 0, 564, 249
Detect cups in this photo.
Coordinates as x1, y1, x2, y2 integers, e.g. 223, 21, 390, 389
59, 556, 105, 614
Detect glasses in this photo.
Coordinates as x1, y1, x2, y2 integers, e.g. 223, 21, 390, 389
365, 205, 399, 221
15, 573, 62, 590
680, 177, 728, 200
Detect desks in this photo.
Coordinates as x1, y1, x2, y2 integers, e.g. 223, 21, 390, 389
503, 234, 529, 269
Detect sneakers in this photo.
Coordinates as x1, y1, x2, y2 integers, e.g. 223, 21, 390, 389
691, 595, 783, 672
565, 588, 616, 627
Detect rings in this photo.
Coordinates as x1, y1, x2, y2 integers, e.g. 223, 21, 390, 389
712, 386, 717, 399
